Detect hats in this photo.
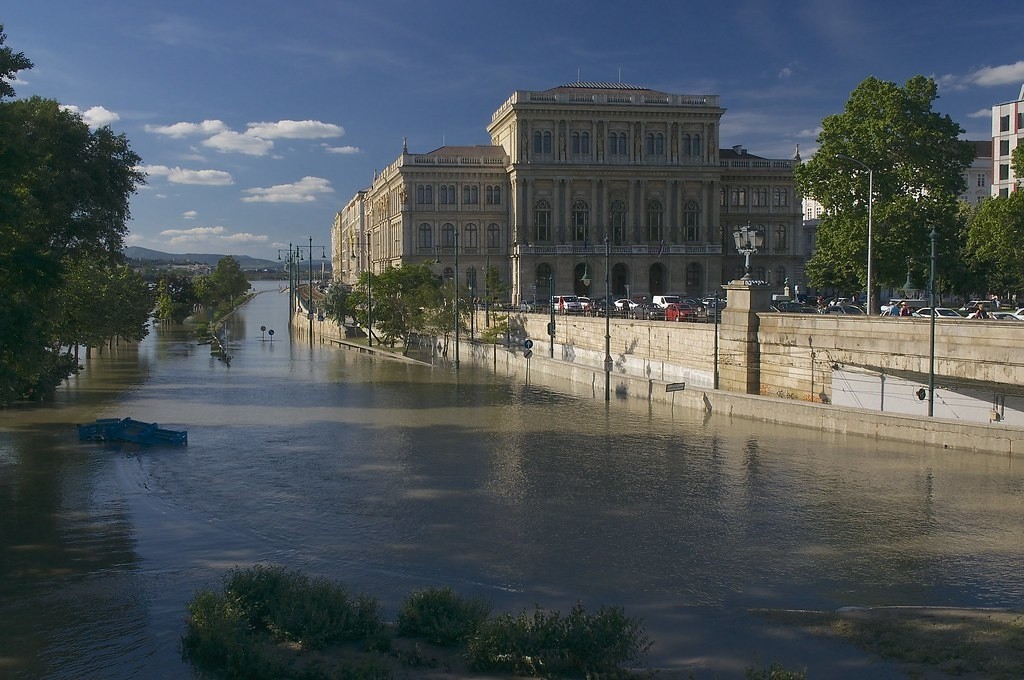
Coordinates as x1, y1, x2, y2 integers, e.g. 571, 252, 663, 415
889, 302, 894, 304
978, 304, 984, 310
901, 302, 907, 306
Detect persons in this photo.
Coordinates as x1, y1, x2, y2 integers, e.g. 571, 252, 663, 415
850, 294, 856, 305
880, 302, 907, 317
975, 304, 994, 319
620, 300, 629, 319
993, 298, 1001, 308
815, 296, 827, 314
802, 298, 807, 307
587, 301, 597, 317
838, 302, 846, 313
1012, 292, 1017, 303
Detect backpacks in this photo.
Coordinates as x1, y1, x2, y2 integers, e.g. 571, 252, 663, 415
979, 312, 987, 319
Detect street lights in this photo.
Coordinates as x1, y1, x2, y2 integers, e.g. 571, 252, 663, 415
431, 229, 461, 368
579, 233, 613, 371
696, 290, 719, 390
350, 229, 372, 347
535, 273, 554, 358
277, 241, 304, 312
900, 225, 937, 419
835, 153, 873, 317
296, 236, 327, 314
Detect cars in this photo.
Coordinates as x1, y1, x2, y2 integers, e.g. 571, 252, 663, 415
548, 291, 726, 322
521, 299, 543, 314
774, 293, 1024, 323
